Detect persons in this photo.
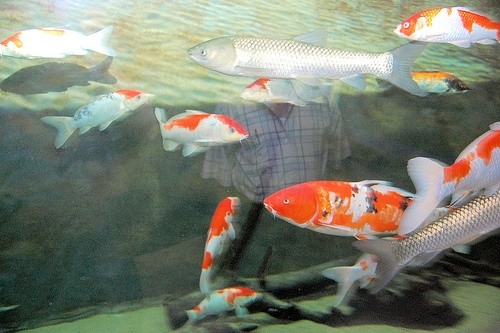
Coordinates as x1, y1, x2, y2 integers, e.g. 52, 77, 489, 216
200, 79, 352, 278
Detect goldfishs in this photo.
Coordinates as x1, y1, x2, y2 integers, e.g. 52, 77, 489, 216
0, 6, 500, 326
